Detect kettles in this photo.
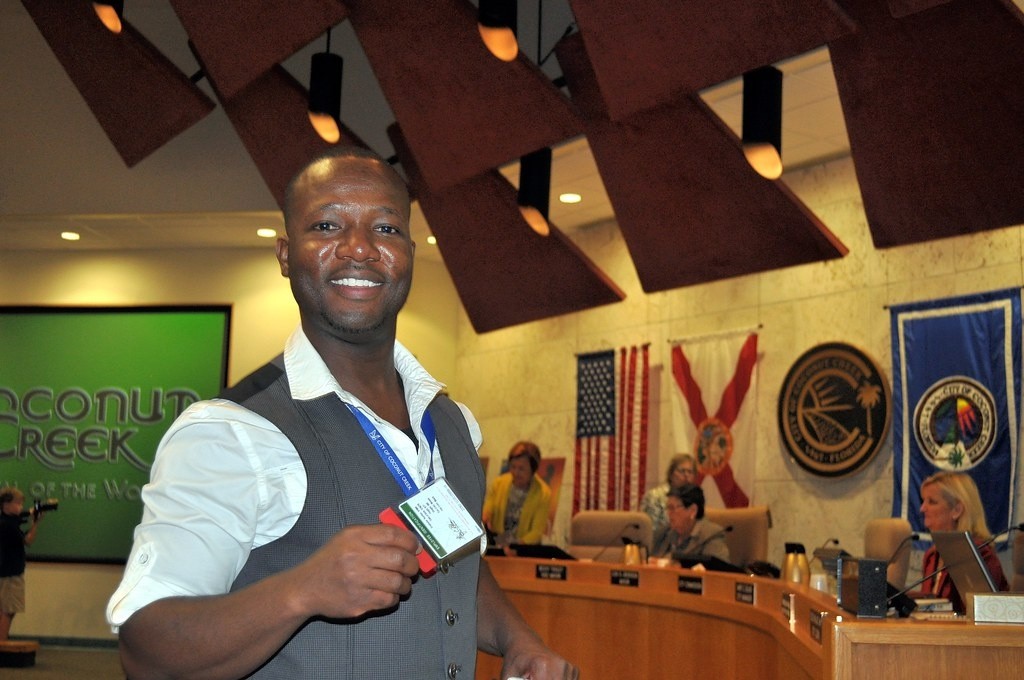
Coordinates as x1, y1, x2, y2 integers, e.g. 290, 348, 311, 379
780, 541, 811, 587
620, 536, 648, 566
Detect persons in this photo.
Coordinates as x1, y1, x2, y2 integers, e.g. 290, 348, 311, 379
638, 454, 699, 525
0, 484, 45, 642
106, 154, 580, 680
655, 482, 732, 563
913, 468, 1011, 613
476, 440, 553, 547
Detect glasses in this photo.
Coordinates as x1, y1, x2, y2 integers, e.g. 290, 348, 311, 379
674, 468, 695, 476
10, 500, 24, 505
665, 504, 683, 511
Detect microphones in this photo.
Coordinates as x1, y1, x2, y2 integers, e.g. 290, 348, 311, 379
887, 523, 1024, 600
685, 525, 733, 555
887, 535, 920, 565
593, 523, 640, 561
808, 538, 840, 567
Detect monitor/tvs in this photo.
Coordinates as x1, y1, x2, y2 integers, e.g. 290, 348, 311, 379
814, 548, 915, 612
672, 552, 744, 572
509, 543, 572, 559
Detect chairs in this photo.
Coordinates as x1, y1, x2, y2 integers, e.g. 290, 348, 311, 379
704, 507, 772, 566
570, 511, 652, 564
864, 518, 911, 591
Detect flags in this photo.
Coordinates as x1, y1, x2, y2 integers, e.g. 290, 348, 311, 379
666, 323, 763, 511
572, 342, 651, 526
883, 286, 1023, 553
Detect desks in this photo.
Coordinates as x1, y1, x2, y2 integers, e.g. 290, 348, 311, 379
476, 556, 1024, 680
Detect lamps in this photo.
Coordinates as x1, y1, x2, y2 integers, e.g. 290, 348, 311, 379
476, 1, 521, 60
95, 0, 126, 34
520, 149, 552, 236
741, 65, 783, 178
308, 29, 344, 143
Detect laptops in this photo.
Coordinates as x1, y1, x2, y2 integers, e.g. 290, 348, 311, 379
933, 530, 1000, 612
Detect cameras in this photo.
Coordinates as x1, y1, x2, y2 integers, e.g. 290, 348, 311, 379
33, 498, 59, 519
